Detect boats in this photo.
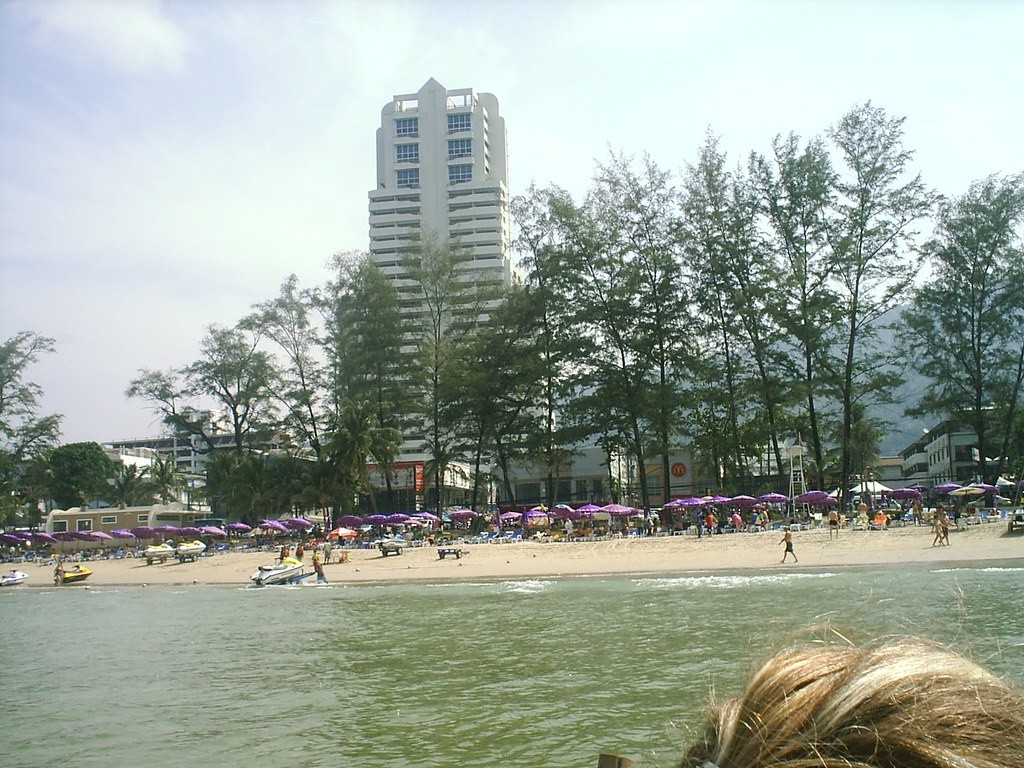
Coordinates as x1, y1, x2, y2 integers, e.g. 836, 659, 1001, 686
178, 540, 207, 555
0, 568, 29, 586
249, 556, 304, 587
144, 543, 177, 559
374, 538, 408, 551
53, 565, 93, 583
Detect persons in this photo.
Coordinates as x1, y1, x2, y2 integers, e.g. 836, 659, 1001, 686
323, 539, 331, 566
682, 624, 1024, 768
312, 549, 323, 580
673, 506, 844, 566
910, 497, 961, 549
853, 497, 890, 532
53, 560, 64, 587
561, 517, 660, 542
280, 543, 304, 561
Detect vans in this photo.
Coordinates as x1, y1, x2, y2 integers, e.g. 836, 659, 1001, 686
853, 494, 901, 511
419, 519, 433, 532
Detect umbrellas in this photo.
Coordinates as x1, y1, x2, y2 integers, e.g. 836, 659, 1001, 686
884, 481, 996, 514
667, 489, 837, 516
91, 530, 113, 548
52, 531, 75, 551
0, 533, 22, 544
178, 516, 314, 542
69, 530, 98, 550
496, 502, 639, 533
109, 524, 178, 547
327, 507, 479, 540
14, 532, 33, 539
34, 533, 59, 545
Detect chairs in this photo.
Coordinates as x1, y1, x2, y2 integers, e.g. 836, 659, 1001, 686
220, 530, 523, 555
40, 551, 134, 565
530, 509, 1010, 543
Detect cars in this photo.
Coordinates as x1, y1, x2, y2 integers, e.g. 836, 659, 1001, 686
630, 510, 659, 526
242, 528, 262, 538
968, 495, 1011, 508
753, 502, 786, 513
354, 524, 373, 535
